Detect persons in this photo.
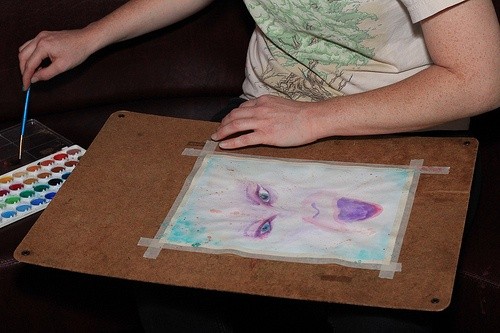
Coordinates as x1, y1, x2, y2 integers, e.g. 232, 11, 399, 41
16, 0, 500, 149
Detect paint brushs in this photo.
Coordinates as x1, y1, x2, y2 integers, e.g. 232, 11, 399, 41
19, 89, 30, 158
